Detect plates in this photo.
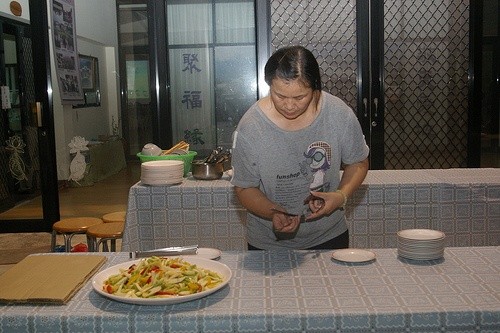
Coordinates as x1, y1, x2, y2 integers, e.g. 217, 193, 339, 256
397, 228, 446, 260
331, 248, 375, 262
196, 247, 221, 259
91, 256, 232, 305
141, 160, 184, 187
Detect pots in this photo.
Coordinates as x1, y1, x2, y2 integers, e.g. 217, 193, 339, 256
192, 160, 224, 180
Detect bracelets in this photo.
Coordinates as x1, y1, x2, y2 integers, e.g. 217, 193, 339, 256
336, 189, 347, 208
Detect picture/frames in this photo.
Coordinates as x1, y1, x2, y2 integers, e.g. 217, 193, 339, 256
72, 53, 102, 108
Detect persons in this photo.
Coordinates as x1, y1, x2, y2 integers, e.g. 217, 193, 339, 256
230, 46, 370, 250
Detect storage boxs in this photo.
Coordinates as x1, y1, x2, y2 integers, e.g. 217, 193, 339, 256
72, 136, 124, 184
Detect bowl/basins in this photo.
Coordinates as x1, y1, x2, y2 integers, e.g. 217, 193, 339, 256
138, 151, 198, 177
141, 143, 162, 156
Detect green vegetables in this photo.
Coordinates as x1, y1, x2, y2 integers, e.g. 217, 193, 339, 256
103, 255, 223, 297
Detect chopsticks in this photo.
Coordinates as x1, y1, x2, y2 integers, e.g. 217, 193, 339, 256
164, 141, 189, 156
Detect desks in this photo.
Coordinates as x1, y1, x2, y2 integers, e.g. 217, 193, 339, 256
0, 251, 500, 333
124, 169, 500, 253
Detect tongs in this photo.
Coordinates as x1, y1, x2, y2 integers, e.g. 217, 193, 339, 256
205, 146, 231, 163
129, 245, 200, 257
269, 208, 306, 233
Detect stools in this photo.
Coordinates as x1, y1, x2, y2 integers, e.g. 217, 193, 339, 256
102, 209, 127, 224
86, 222, 126, 252
50, 216, 103, 253
